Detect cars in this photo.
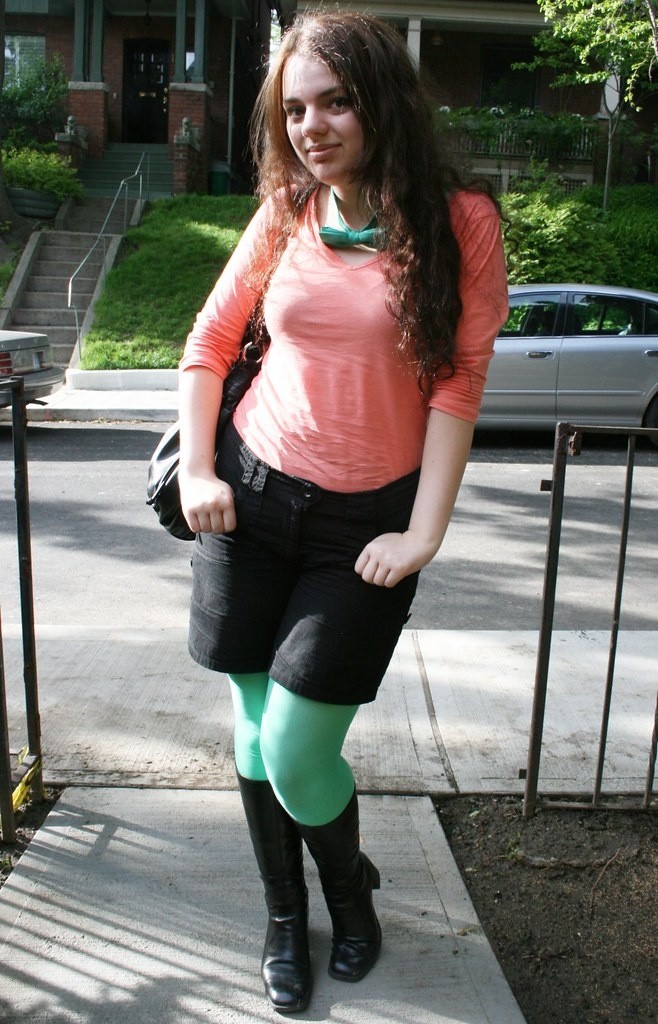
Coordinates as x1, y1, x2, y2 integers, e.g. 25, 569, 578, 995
0, 328, 67, 411
470, 280, 657, 456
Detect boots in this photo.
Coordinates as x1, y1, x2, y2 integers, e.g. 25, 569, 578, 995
235, 763, 311, 1011
294, 782, 381, 983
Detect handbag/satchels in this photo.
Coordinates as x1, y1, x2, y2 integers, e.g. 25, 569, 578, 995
144, 181, 327, 539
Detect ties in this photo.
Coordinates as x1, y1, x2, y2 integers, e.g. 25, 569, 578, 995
318, 190, 390, 246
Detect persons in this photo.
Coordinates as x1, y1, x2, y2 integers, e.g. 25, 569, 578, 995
179, 13, 509, 1013
